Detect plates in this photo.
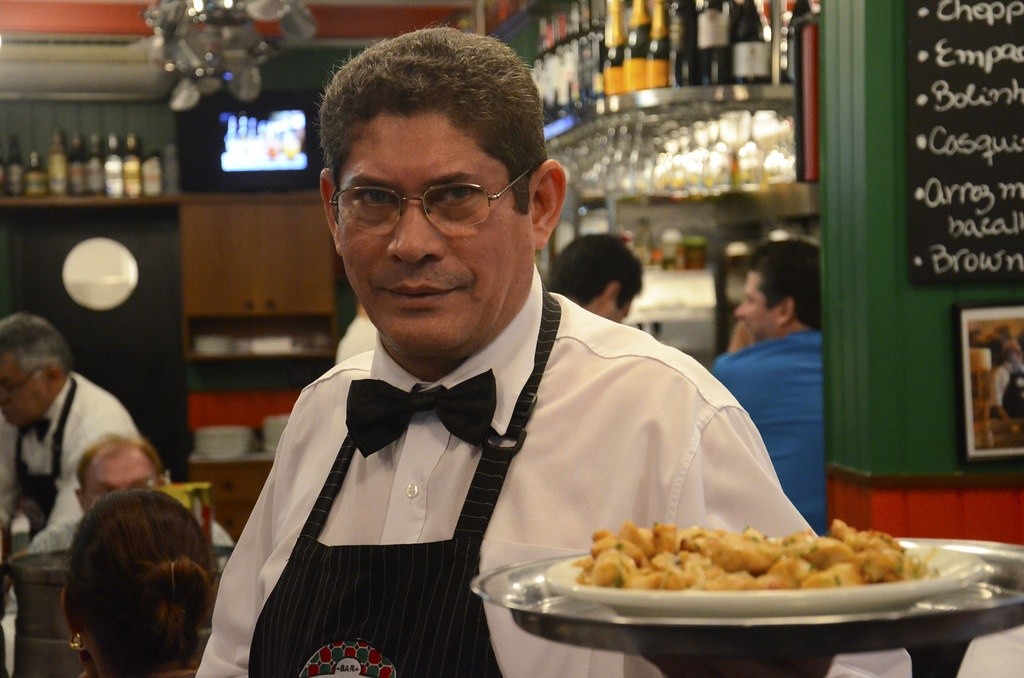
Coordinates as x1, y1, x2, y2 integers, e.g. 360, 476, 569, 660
194, 336, 293, 354
263, 414, 292, 453
193, 425, 254, 460
545, 548, 987, 616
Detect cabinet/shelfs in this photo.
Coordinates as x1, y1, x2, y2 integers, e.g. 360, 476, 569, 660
185, 454, 275, 543
180, 192, 342, 363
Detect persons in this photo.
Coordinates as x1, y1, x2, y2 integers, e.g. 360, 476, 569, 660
193, 27, 912, 678
0, 315, 141, 601
972, 324, 1024, 446
61, 490, 214, 678
551, 232, 643, 323
76, 437, 166, 510
712, 239, 827, 536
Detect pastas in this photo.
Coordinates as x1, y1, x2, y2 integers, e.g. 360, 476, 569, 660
570, 517, 925, 591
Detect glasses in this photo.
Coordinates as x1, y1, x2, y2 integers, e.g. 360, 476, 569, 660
329, 167, 532, 236
0, 366, 45, 407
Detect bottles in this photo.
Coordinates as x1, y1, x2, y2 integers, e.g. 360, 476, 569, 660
530, 0, 812, 124
577, 186, 708, 273
0, 128, 182, 196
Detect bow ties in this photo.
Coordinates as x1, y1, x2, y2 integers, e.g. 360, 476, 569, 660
346, 368, 497, 458
19, 418, 49, 441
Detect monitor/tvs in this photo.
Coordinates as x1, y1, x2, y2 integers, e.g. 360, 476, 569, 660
180, 86, 329, 195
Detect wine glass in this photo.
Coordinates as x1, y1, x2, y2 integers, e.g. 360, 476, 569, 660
549, 109, 798, 195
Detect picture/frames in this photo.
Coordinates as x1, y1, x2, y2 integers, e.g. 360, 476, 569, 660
955, 301, 1024, 465
